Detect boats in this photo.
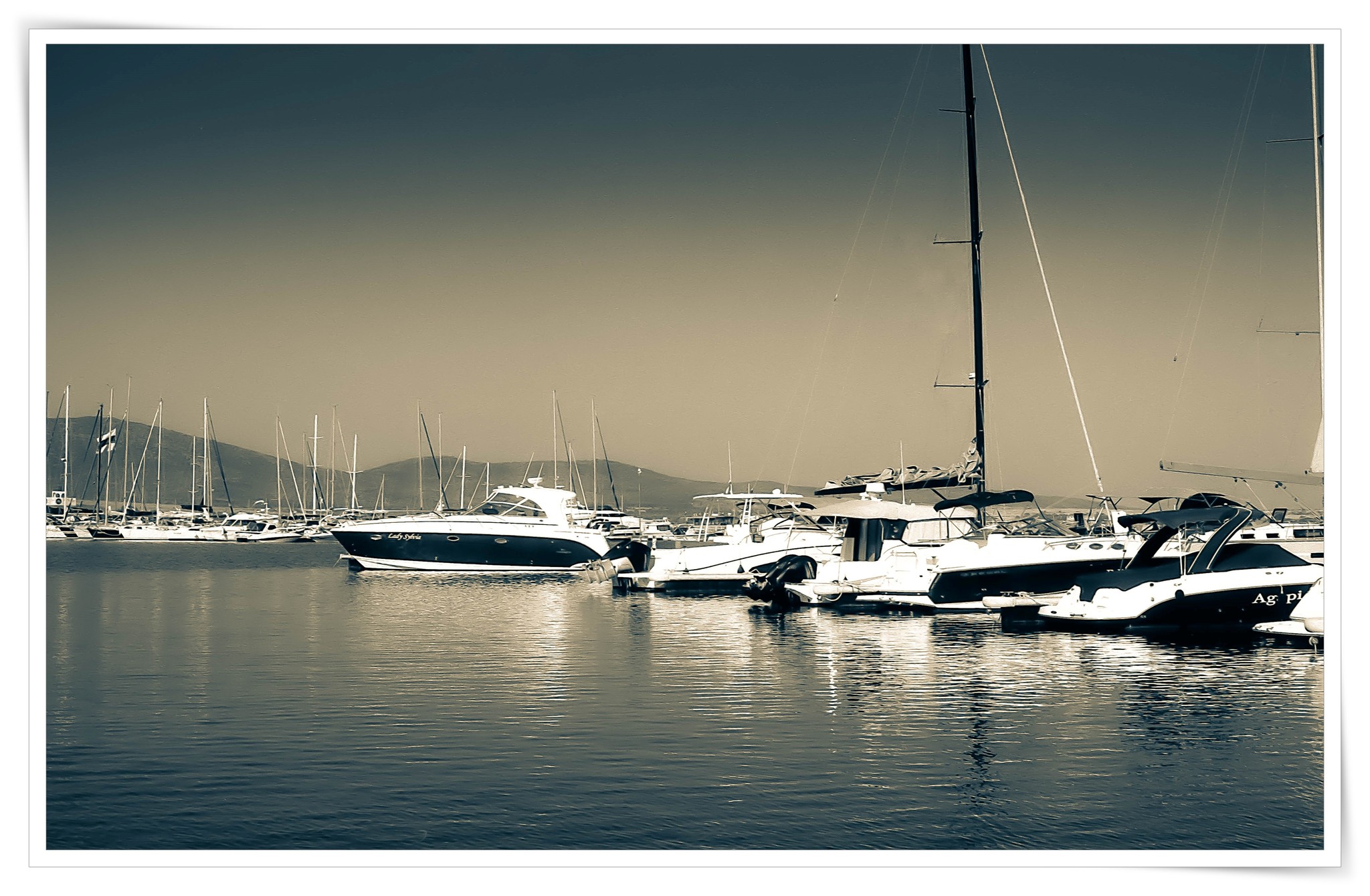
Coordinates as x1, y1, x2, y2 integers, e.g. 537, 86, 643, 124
609, 44, 1323, 640
329, 475, 614, 578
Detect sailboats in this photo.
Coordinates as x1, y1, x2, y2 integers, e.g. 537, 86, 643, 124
46, 376, 681, 544
